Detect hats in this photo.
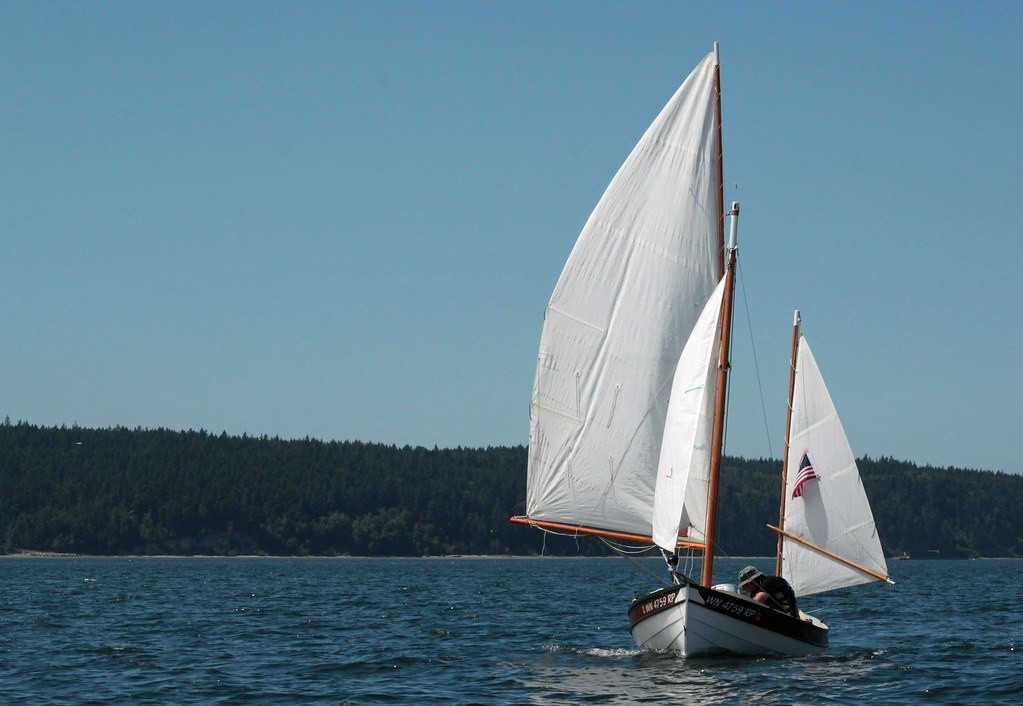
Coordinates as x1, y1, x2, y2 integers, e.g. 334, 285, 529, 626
738, 566, 763, 588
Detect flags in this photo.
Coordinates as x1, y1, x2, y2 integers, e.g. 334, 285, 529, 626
791, 447, 822, 500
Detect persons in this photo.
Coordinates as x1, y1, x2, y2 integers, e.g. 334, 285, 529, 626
738, 565, 799, 619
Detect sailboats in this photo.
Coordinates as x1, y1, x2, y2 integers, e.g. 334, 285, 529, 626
506, 41, 898, 661
